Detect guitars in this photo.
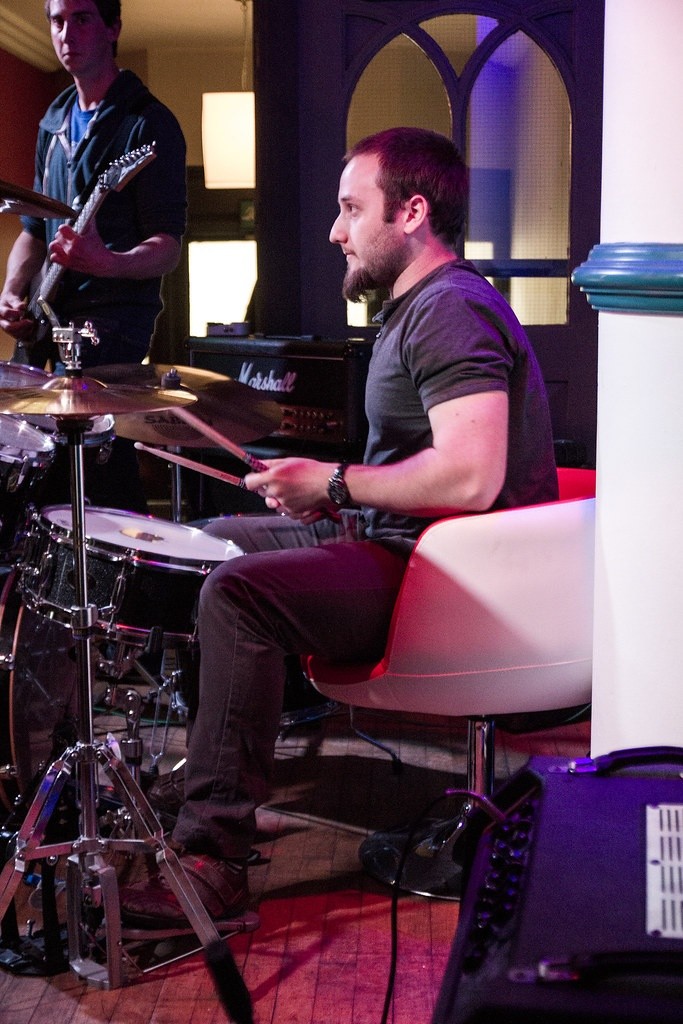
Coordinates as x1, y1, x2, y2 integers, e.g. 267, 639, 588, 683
21, 137, 163, 348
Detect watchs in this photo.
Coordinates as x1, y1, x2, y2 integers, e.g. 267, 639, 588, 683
324, 463, 354, 511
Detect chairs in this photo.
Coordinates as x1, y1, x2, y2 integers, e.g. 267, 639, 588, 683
302, 464, 597, 908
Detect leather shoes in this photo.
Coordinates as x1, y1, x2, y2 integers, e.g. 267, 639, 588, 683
119, 853, 250, 925
98, 768, 182, 823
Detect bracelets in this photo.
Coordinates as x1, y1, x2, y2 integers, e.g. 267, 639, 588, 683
113, 249, 128, 277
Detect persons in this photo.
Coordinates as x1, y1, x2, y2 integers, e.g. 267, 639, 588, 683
0, 0, 184, 720
77, 125, 556, 922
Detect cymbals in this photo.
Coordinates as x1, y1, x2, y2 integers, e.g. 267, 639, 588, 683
0, 376, 201, 420
76, 363, 283, 449
0, 178, 79, 224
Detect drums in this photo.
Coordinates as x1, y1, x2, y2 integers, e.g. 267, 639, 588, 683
16, 501, 248, 656
0, 357, 117, 478
0, 411, 57, 555
